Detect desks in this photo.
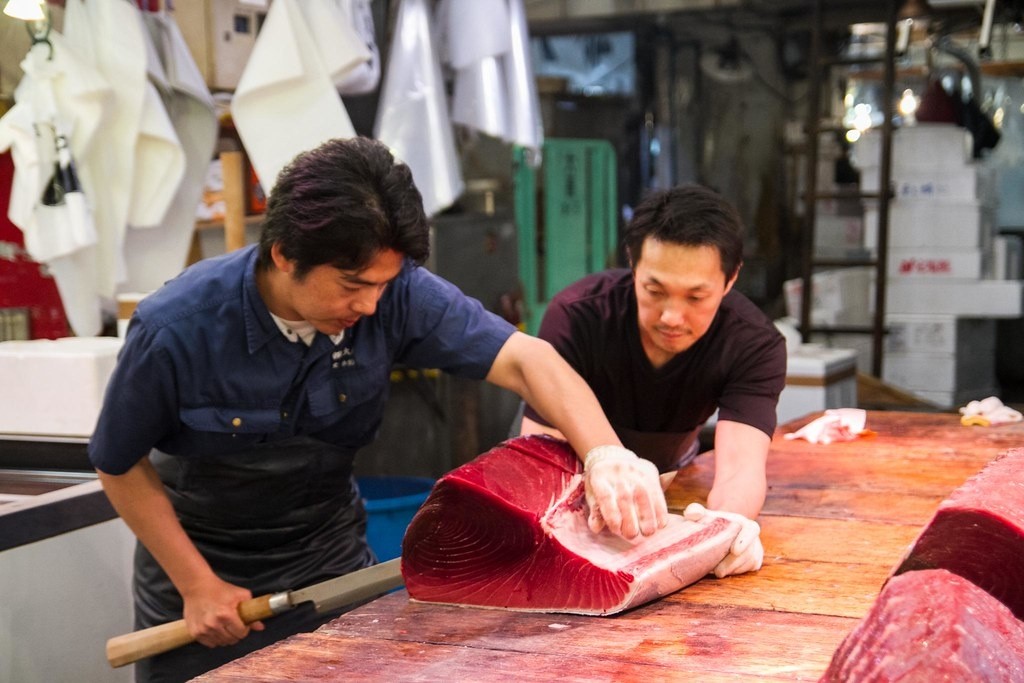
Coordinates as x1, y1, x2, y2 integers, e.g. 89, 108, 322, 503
180, 406, 1024, 680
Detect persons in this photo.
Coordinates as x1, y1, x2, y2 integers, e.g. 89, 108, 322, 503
87, 135, 669, 683
509, 184, 787, 578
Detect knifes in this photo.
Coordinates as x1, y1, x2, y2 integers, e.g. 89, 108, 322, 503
105, 556, 406, 668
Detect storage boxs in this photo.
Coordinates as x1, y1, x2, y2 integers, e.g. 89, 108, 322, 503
703, 120, 1022, 427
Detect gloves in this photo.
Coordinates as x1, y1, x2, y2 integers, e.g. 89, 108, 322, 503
582, 443, 669, 540
683, 500, 765, 578
